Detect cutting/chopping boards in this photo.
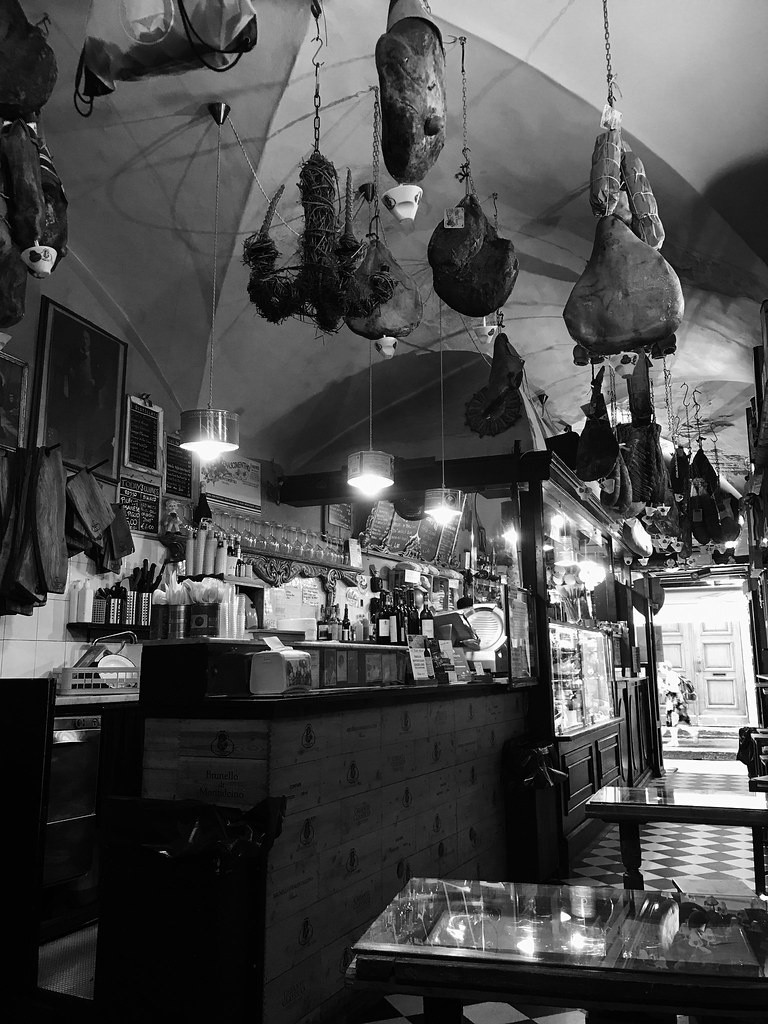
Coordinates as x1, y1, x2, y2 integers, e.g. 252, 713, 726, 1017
1, 445, 135, 618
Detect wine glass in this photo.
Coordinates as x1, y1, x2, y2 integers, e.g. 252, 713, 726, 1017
210, 507, 349, 565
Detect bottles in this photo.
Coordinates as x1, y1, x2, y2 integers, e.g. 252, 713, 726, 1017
317, 602, 434, 646
424, 640, 434, 679
218, 531, 254, 579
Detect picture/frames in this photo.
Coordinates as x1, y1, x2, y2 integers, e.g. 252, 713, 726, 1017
26, 295, 129, 487
0, 351, 30, 454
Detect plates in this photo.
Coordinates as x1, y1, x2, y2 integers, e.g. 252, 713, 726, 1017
98, 654, 136, 689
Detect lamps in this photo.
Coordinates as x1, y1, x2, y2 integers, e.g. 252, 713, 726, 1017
424, 297, 464, 529
501, 498, 606, 584
180, 104, 240, 462
347, 182, 396, 497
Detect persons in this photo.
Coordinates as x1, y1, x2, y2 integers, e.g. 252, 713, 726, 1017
658, 661, 693, 729
0, 330, 101, 442
163, 499, 183, 536
457, 598, 476, 653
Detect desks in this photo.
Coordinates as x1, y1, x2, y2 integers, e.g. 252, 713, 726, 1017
586, 785, 768, 896
343, 877, 768, 1024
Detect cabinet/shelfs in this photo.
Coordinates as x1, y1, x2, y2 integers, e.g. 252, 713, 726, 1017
547, 617, 626, 866
